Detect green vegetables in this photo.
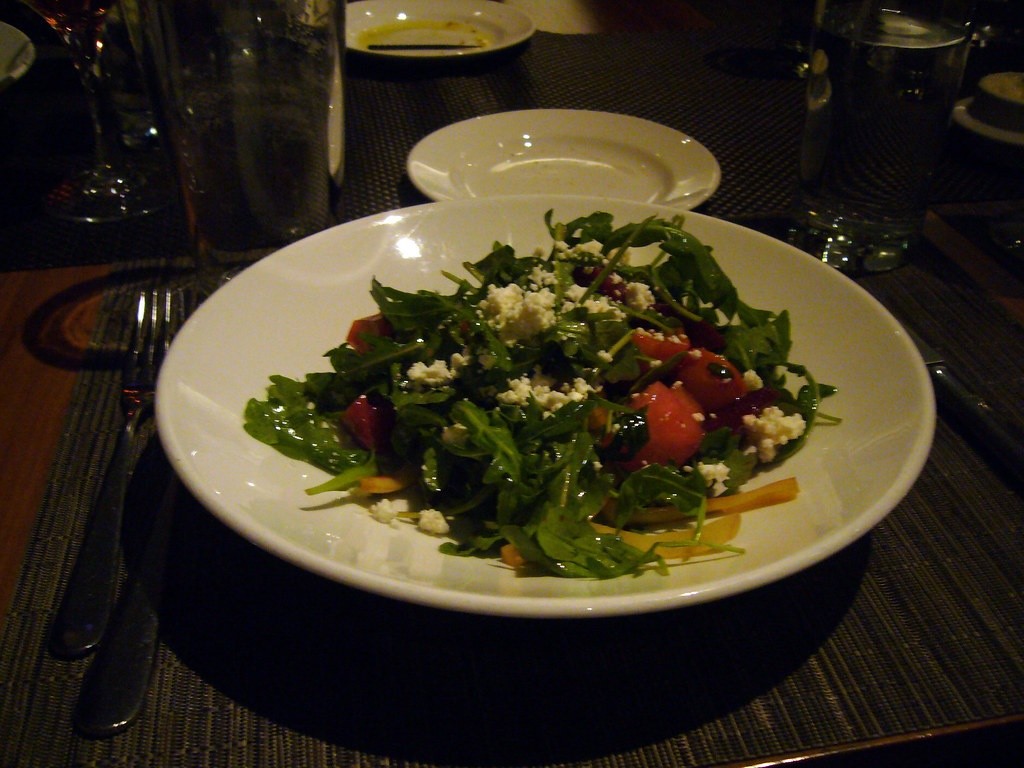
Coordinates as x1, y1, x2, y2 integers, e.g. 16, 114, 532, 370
243, 208, 840, 578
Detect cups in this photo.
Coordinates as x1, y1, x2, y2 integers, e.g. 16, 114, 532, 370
118, 0, 346, 299
786, 0, 979, 271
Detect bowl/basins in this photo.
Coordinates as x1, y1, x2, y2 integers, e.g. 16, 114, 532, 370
977, 71, 1024, 134
0, 21, 35, 89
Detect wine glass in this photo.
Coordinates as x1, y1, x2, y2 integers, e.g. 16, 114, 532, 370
21, 0, 164, 224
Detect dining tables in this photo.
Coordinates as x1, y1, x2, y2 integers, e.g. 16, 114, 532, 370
1, 0, 1024, 768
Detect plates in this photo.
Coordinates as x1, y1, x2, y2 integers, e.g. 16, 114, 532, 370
407, 109, 721, 212
951, 96, 1024, 146
154, 193, 937, 617
344, 0, 537, 58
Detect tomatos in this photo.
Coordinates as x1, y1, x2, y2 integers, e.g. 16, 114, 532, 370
338, 392, 379, 450
626, 382, 708, 467
625, 327, 689, 372
676, 346, 752, 408
345, 313, 392, 353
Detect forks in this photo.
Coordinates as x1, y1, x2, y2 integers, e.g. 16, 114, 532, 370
46, 287, 186, 655
